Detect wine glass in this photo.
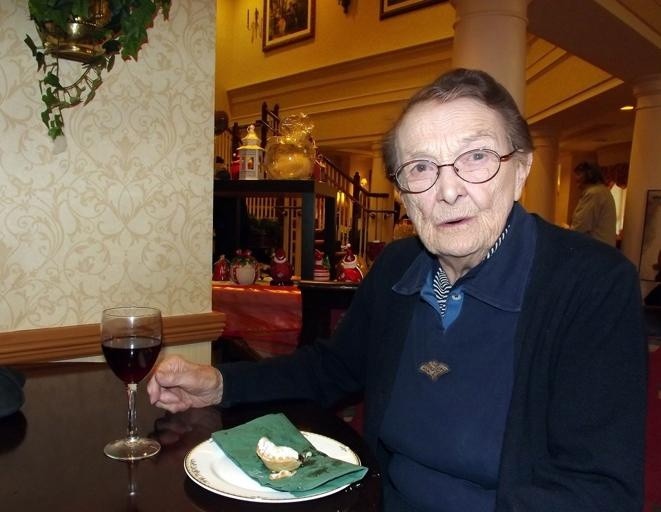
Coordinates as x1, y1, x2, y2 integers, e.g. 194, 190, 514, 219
99, 304, 163, 464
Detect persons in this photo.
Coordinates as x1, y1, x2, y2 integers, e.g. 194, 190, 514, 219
571, 159, 616, 248
146, 69, 647, 512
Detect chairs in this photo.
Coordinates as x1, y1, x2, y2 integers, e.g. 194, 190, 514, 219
296, 280, 357, 348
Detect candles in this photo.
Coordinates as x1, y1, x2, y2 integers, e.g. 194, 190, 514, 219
245, 9, 249, 29
254, 9, 258, 27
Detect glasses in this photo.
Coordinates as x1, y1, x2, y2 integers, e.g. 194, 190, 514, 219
385, 146, 521, 194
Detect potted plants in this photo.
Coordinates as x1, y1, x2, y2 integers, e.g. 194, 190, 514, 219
16, 0, 175, 138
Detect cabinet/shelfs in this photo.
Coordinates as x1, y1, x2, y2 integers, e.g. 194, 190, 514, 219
212, 178, 361, 423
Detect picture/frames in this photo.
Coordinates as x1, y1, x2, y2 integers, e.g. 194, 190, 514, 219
260, 0, 316, 52
636, 189, 660, 284
378, 0, 442, 21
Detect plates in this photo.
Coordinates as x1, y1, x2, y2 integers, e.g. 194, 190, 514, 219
183, 428, 362, 507
